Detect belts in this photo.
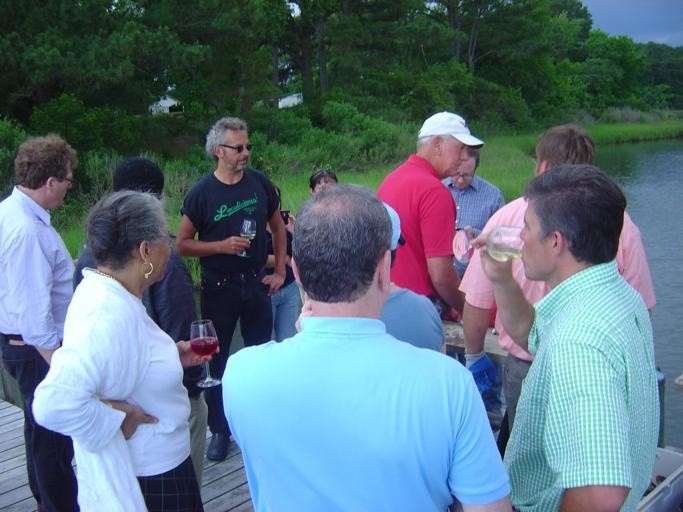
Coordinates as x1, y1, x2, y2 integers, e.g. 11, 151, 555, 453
201, 272, 265, 289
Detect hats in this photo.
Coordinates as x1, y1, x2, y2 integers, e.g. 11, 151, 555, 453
418, 111, 484, 149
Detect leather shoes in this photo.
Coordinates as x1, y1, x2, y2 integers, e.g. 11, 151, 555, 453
207, 433, 231, 462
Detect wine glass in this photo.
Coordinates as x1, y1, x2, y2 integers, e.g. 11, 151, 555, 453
188, 318, 225, 390
451, 226, 526, 267
234, 216, 259, 258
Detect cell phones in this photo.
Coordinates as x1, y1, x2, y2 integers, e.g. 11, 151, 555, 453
280, 210, 289, 226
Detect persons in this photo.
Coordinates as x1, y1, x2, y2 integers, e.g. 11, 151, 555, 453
0, 159, 210, 512
224, 184, 514, 512
175, 111, 656, 466
465, 164, 662, 512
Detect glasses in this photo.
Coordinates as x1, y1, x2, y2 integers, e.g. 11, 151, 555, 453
64, 178, 74, 183
219, 144, 252, 153
452, 175, 475, 180
150, 234, 176, 246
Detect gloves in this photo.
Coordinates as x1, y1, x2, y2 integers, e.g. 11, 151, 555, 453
465, 350, 503, 394
